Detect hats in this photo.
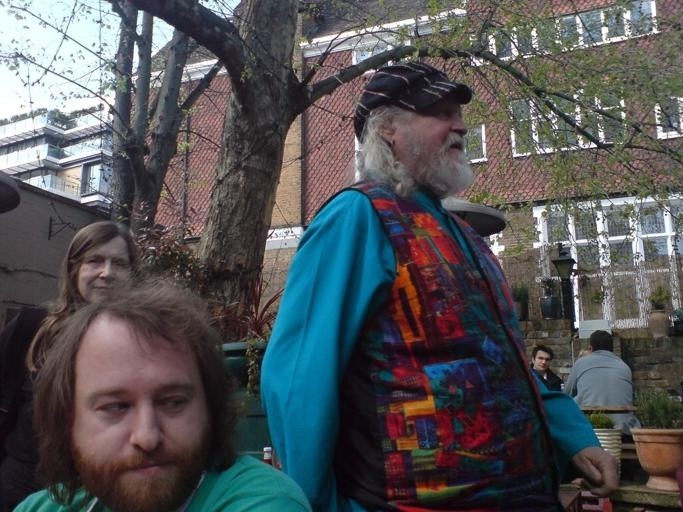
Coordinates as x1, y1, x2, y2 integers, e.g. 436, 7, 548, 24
350, 57, 475, 143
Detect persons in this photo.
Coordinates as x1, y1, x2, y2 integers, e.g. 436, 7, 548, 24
0, 219, 145, 511
563, 329, 640, 429
7, 285, 316, 512
529, 342, 566, 393
256, 58, 619, 511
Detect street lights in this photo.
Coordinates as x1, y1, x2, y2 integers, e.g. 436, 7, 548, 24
550, 239, 579, 368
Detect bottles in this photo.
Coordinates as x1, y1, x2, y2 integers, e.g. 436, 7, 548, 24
262, 446, 274, 470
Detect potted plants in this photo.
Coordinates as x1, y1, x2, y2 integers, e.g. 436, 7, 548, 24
536, 275, 562, 321
647, 282, 674, 340
509, 281, 529, 321
586, 410, 622, 480
626, 387, 682, 492
